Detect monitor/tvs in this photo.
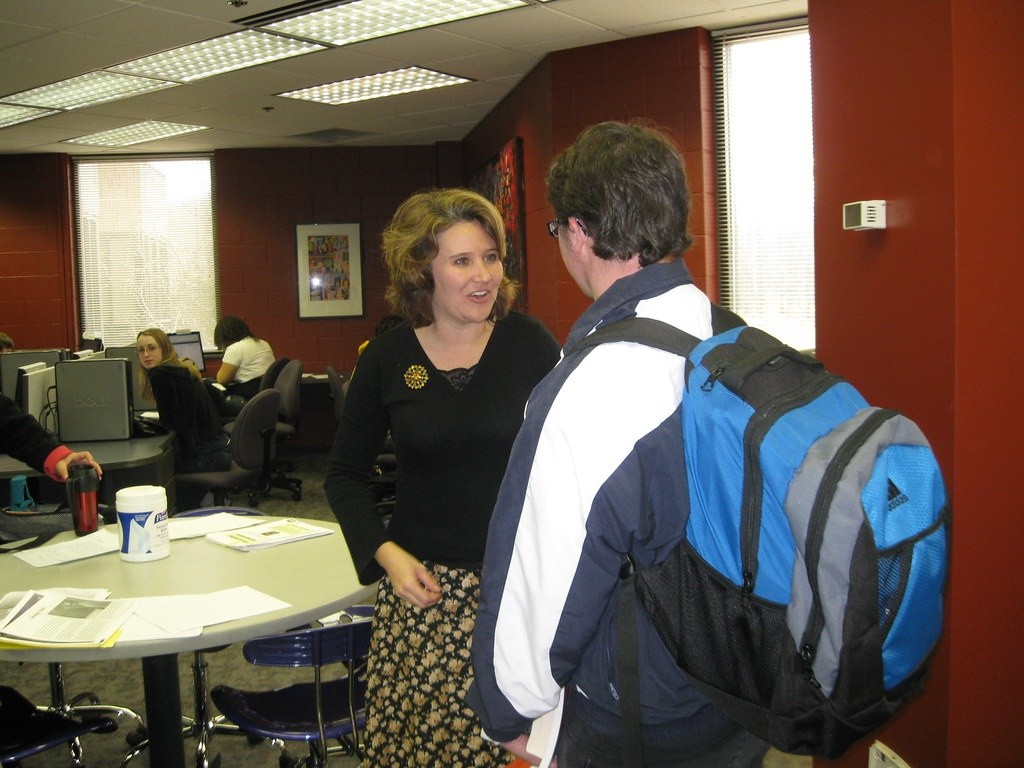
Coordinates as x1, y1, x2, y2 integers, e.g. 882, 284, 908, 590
166, 332, 210, 371
15, 361, 46, 404
73, 349, 94, 359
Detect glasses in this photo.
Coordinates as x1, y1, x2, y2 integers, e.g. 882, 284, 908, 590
546, 215, 589, 239
135, 344, 160, 355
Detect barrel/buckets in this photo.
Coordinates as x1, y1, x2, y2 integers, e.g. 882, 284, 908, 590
115, 484, 170, 563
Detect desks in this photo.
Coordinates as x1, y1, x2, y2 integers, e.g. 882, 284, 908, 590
0, 345, 342, 513
0, 516, 382, 767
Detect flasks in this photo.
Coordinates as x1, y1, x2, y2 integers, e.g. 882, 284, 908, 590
67, 464, 98, 535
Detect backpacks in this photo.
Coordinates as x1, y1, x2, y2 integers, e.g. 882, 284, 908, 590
561, 300, 951, 760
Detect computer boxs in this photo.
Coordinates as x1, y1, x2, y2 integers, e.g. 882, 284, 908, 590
105, 346, 158, 410
54, 358, 133, 443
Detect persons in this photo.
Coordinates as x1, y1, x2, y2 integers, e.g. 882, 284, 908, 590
347, 314, 396, 453
137, 329, 232, 475
322, 189, 561, 767
0, 393, 103, 484
464, 121, 772, 767
214, 317, 276, 399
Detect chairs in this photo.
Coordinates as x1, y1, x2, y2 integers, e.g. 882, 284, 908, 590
177, 357, 346, 513
0, 602, 385, 768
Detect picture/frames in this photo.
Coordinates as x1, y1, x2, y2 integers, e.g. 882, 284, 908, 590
292, 222, 365, 319
452, 136, 529, 315
